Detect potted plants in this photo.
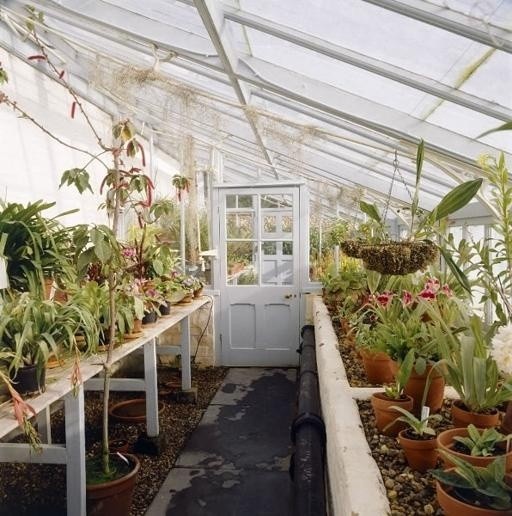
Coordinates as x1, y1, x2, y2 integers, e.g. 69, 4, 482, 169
0, 219, 204, 397
57, 117, 175, 516
